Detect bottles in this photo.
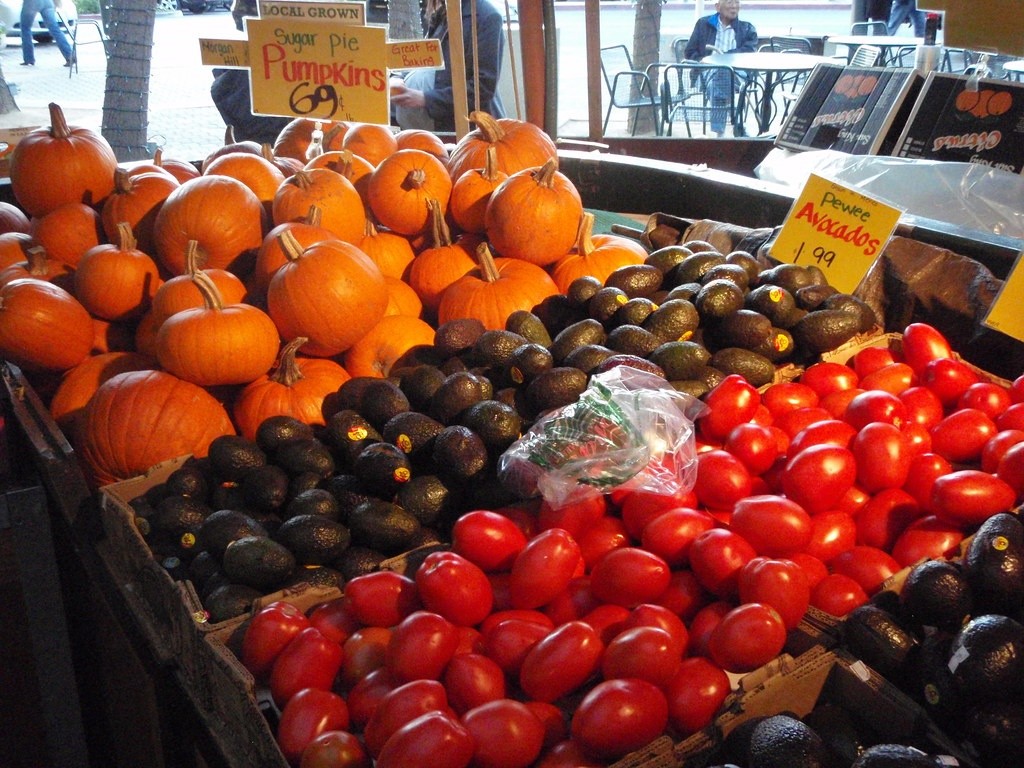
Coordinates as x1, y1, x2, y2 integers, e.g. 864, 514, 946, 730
923, 14, 938, 45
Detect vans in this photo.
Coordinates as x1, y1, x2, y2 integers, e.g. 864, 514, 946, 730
0, 0, 79, 45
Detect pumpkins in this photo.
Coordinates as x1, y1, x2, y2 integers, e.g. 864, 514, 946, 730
0, 104, 646, 486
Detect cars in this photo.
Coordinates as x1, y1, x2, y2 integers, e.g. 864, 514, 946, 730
157, 0, 234, 14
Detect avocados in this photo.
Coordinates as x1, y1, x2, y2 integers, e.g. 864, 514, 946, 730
700, 512, 1024, 768
130, 239, 877, 622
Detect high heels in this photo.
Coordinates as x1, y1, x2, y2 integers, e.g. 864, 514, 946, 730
20, 62, 34, 66
63, 59, 77, 67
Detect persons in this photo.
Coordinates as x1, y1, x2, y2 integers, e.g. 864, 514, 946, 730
21, 0, 77, 67
210, 0, 504, 148
847, 0, 942, 66
684, 0, 758, 137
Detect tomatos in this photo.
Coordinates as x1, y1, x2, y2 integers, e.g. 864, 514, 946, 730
241, 440, 811, 768
696, 324, 1024, 615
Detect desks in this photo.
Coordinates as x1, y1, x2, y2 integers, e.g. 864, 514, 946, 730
1001, 61, 1024, 83
703, 52, 839, 129
828, 36, 941, 65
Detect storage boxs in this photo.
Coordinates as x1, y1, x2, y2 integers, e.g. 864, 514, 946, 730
0, 321, 1024, 768
774, 61, 926, 157
891, 70, 1024, 175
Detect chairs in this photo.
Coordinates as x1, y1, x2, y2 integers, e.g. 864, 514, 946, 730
598, 22, 1024, 139
53, 10, 109, 77
